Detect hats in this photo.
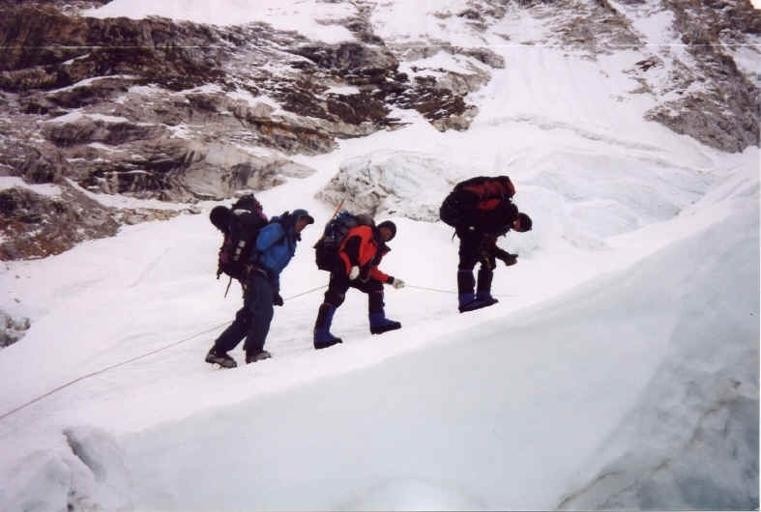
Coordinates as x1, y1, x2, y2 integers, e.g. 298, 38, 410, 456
519, 213, 532, 232
291, 209, 314, 225
377, 221, 396, 241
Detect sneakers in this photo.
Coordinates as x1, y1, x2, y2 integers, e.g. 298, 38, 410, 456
205, 345, 237, 368
246, 351, 271, 364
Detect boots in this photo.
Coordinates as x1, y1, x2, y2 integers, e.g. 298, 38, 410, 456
313, 302, 342, 349
369, 291, 401, 334
457, 269, 498, 313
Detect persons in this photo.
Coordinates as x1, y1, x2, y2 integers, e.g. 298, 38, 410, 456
437, 176, 532, 312
205, 193, 402, 370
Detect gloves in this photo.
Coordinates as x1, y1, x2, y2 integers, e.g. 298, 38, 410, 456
500, 251, 518, 266
392, 279, 405, 289
273, 294, 284, 306
349, 266, 359, 280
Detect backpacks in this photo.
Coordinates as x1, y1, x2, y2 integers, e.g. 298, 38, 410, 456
439, 175, 517, 226
314, 211, 376, 272
210, 193, 287, 282
